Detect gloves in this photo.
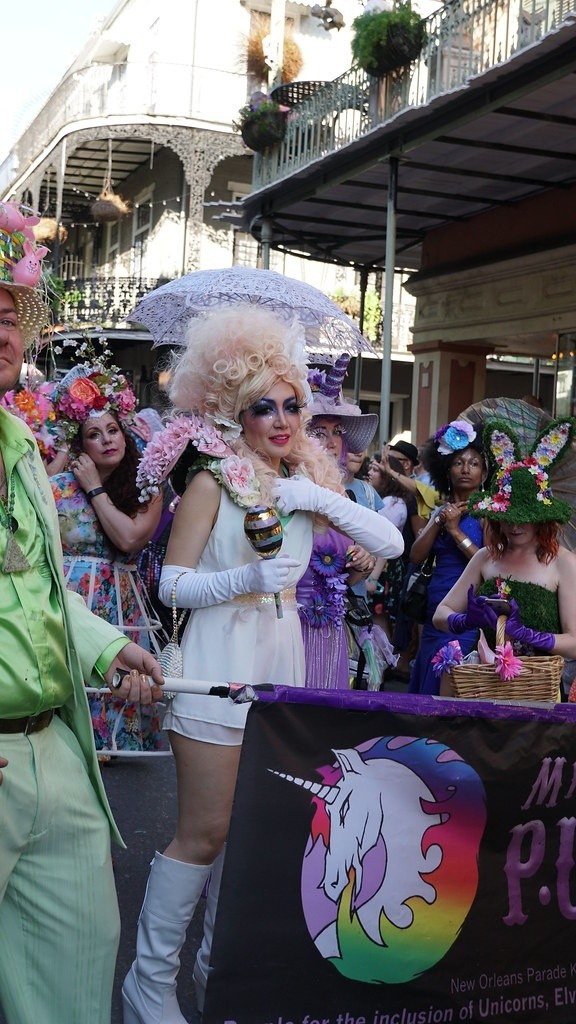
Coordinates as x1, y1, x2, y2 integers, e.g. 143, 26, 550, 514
448, 584, 500, 634
481, 599, 556, 652
158, 555, 301, 609
270, 474, 405, 559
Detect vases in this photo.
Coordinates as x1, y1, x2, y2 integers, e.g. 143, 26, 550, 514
239, 111, 286, 156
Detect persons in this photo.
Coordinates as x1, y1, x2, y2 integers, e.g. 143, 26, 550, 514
121, 303, 405, 1024
0, 203, 128, 1024
408, 420, 576, 705
0, 360, 193, 763
347, 440, 450, 675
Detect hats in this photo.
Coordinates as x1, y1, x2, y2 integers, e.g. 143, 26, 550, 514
306, 350, 380, 453
468, 414, 576, 523
389, 440, 420, 467
0, 199, 46, 352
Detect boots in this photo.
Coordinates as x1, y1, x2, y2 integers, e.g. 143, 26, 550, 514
192, 843, 237, 1024
122, 848, 218, 1024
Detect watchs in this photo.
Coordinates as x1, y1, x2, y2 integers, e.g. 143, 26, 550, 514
86, 487, 107, 500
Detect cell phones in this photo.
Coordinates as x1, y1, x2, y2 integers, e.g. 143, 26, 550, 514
486, 599, 513, 616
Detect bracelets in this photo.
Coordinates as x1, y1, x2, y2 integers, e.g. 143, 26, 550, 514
457, 537, 473, 551
393, 472, 400, 482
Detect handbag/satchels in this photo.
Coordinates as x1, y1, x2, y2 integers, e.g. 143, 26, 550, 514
154, 571, 192, 700
403, 566, 433, 625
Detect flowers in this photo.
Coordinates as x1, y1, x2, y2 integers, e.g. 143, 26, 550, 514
305, 546, 350, 628
11, 326, 264, 509
495, 575, 511, 601
436, 421, 570, 513
231, 99, 280, 132
495, 641, 521, 678
433, 642, 464, 671
307, 369, 327, 394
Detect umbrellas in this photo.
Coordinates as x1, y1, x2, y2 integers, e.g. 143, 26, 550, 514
128, 267, 380, 361
451, 397, 576, 553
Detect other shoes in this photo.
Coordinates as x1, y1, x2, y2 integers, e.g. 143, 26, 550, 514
392, 666, 411, 684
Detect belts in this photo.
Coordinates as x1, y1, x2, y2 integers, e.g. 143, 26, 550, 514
0, 709, 55, 736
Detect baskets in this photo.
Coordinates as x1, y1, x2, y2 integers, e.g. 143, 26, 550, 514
449, 618, 564, 711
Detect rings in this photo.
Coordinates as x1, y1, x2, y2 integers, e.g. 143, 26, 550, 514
449, 509, 451, 512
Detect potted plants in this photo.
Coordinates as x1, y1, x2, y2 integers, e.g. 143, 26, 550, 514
349, 3, 429, 78
327, 284, 386, 346
